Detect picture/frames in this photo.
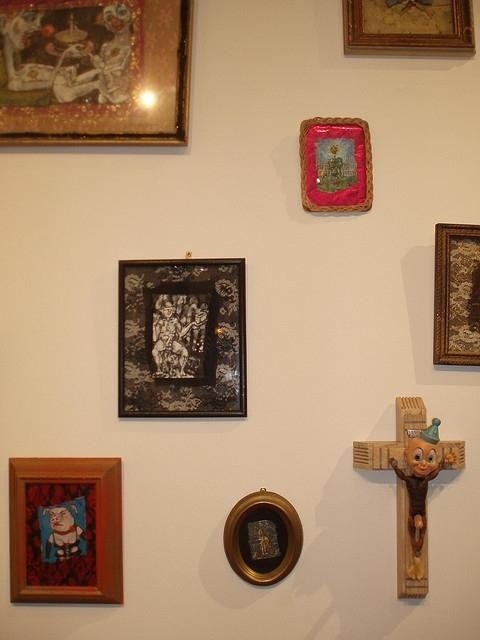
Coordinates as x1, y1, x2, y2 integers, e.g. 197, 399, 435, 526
0, 1, 190, 149
114, 258, 249, 420
296, 115, 376, 216
7, 456, 126, 610
222, 492, 306, 589
429, 220, 480, 369
341, 1, 479, 61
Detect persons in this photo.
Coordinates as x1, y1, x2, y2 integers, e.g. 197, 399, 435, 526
150, 299, 206, 376
388, 417, 456, 556
